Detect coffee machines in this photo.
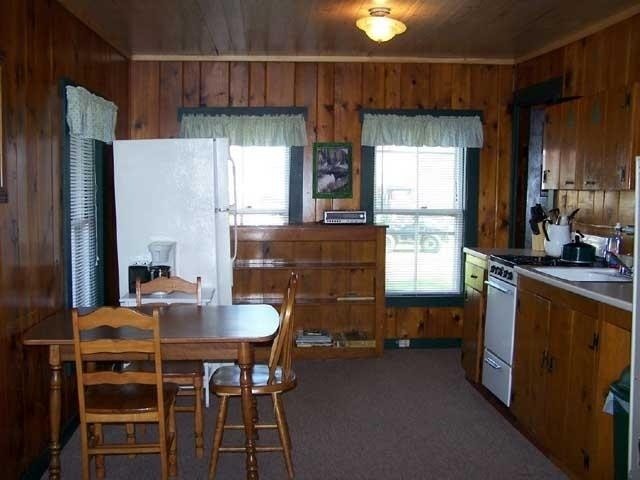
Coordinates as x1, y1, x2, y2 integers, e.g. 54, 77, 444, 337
128, 241, 176, 296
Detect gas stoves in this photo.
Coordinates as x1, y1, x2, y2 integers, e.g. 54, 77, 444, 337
488, 233, 617, 284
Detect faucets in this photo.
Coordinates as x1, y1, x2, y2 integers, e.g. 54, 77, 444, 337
603, 250, 635, 280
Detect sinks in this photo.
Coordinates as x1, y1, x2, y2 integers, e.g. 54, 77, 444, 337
535, 262, 631, 282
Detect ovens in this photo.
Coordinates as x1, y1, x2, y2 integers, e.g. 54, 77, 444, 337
480, 276, 518, 408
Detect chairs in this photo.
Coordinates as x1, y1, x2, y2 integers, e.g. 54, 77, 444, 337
120, 276, 205, 461
71, 305, 178, 480
355, 7, 407, 42
208, 270, 299, 480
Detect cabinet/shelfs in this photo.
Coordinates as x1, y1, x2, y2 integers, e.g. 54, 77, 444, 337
485, 255, 517, 409
541, 82, 640, 191
230, 224, 389, 360
570, 301, 633, 480
517, 271, 568, 480
461, 245, 488, 386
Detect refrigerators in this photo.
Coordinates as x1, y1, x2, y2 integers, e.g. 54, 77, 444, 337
113, 137, 238, 388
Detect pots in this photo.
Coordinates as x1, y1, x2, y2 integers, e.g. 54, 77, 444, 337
560, 230, 596, 265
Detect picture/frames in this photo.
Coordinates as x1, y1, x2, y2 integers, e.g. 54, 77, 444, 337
313, 142, 353, 199
